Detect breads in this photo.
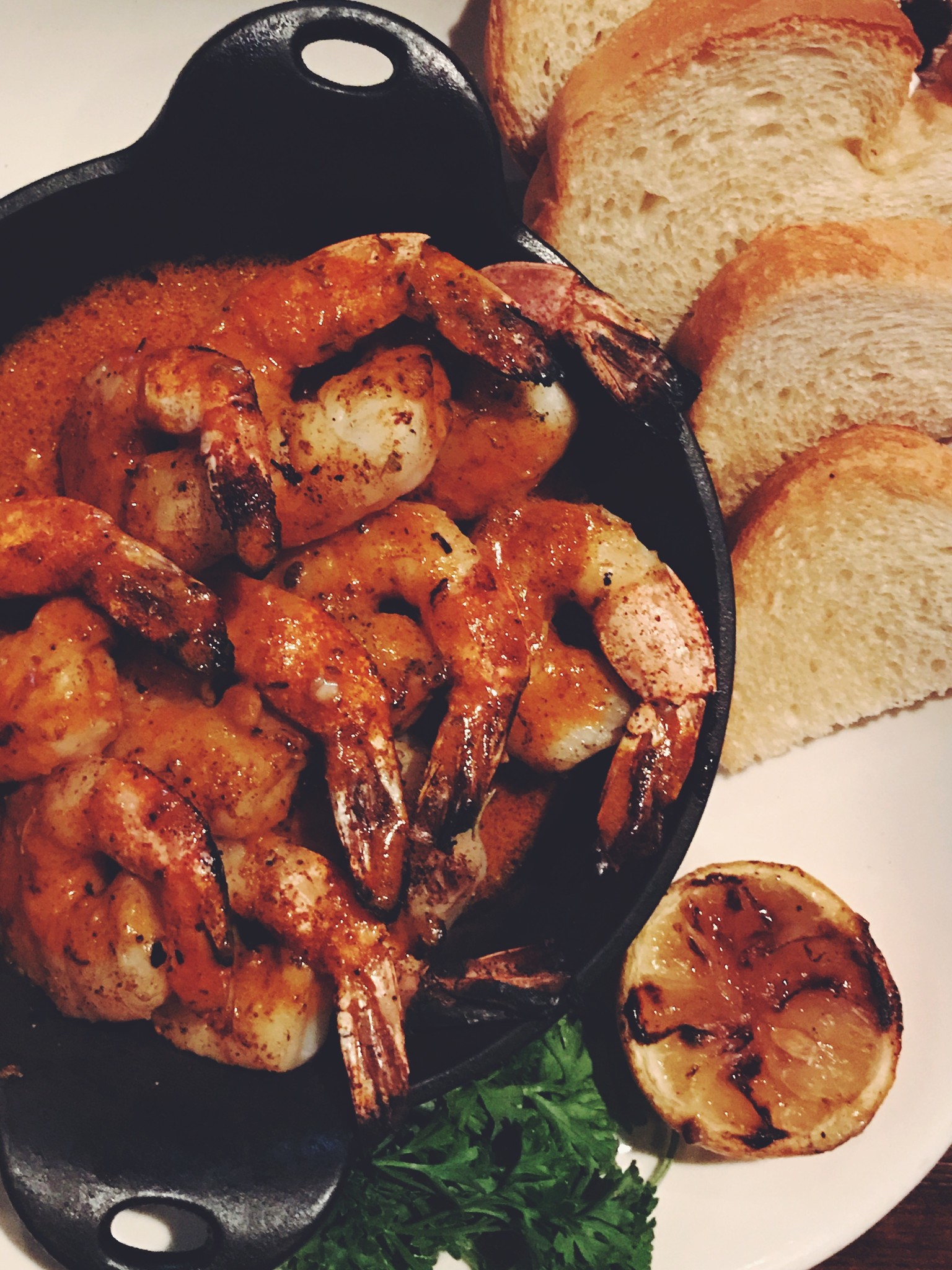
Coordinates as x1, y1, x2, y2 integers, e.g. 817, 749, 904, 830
491, 0, 952, 774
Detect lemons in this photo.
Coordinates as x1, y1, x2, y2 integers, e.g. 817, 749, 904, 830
614, 859, 903, 1160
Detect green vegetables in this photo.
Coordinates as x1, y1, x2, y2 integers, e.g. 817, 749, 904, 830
265, 1009, 681, 1270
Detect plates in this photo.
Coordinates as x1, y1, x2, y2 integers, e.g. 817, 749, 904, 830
424, 697, 952, 1270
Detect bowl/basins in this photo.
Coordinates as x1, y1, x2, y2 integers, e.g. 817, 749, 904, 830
0, 0, 741, 1270
